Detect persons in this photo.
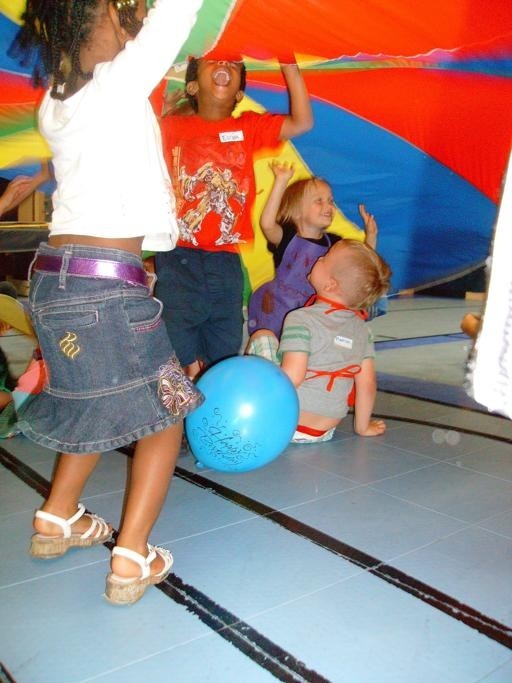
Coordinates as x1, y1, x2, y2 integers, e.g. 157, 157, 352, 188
0, 159, 50, 391
9, 1, 206, 607
246, 159, 392, 445
153, 54, 313, 367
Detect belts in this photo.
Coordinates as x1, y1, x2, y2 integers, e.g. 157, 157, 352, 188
34, 253, 150, 295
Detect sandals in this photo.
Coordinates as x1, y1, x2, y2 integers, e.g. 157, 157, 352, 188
104, 538, 174, 607
25, 506, 113, 560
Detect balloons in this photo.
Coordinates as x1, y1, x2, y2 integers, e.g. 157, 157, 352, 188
183, 352, 303, 475
